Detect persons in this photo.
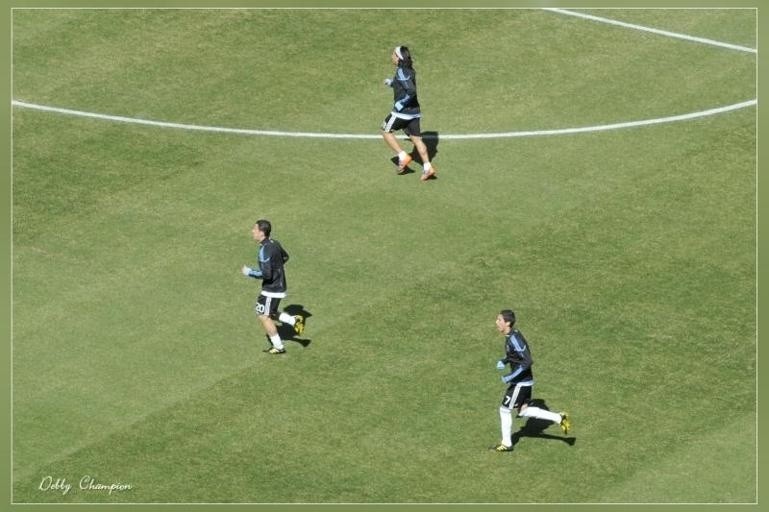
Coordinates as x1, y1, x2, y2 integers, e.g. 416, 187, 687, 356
489, 309, 570, 452
240, 219, 304, 355
380, 45, 436, 182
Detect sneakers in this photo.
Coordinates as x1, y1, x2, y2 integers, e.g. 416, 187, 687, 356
294, 315, 304, 337
269, 346, 287, 354
489, 443, 513, 453
559, 411, 571, 435
397, 155, 411, 175
420, 168, 435, 180
